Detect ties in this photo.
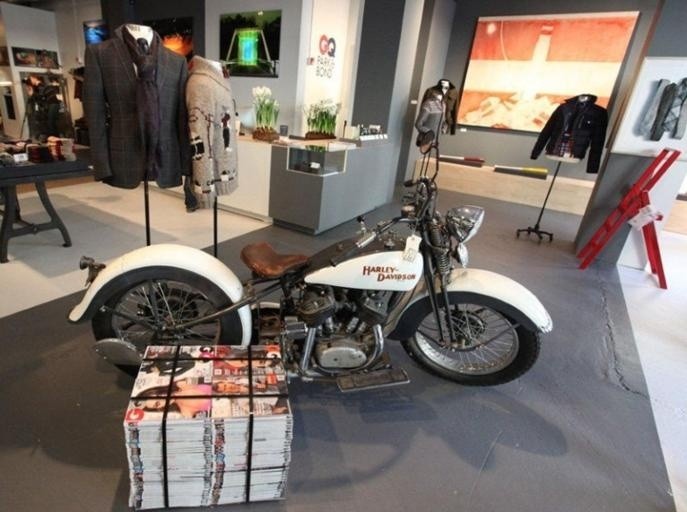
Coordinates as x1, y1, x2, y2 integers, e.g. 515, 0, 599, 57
137, 37, 150, 56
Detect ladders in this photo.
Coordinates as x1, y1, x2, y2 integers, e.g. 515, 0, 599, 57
577, 147, 682, 290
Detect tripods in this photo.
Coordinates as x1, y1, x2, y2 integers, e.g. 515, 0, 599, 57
19, 96, 34, 138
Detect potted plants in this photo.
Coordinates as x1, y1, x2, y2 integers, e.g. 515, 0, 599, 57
252, 86, 280, 142
306, 101, 341, 139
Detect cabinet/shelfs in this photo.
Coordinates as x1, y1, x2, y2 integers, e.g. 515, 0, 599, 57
0, 40, 64, 142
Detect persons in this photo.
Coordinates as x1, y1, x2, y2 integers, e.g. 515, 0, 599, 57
81, 22, 191, 189
530, 93, 609, 174
184, 54, 238, 199
26, 74, 60, 136
420, 77, 460, 136
416, 93, 449, 146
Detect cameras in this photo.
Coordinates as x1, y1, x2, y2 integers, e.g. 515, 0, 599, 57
22, 77, 31, 86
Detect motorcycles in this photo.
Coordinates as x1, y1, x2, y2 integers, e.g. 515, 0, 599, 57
68, 130, 553, 393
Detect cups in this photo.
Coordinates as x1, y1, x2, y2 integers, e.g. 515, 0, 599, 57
280, 125, 288, 136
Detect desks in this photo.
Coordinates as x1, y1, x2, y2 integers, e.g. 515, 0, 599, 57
0, 159, 96, 262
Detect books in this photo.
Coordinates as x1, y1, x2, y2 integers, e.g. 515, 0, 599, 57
122, 342, 294, 511
